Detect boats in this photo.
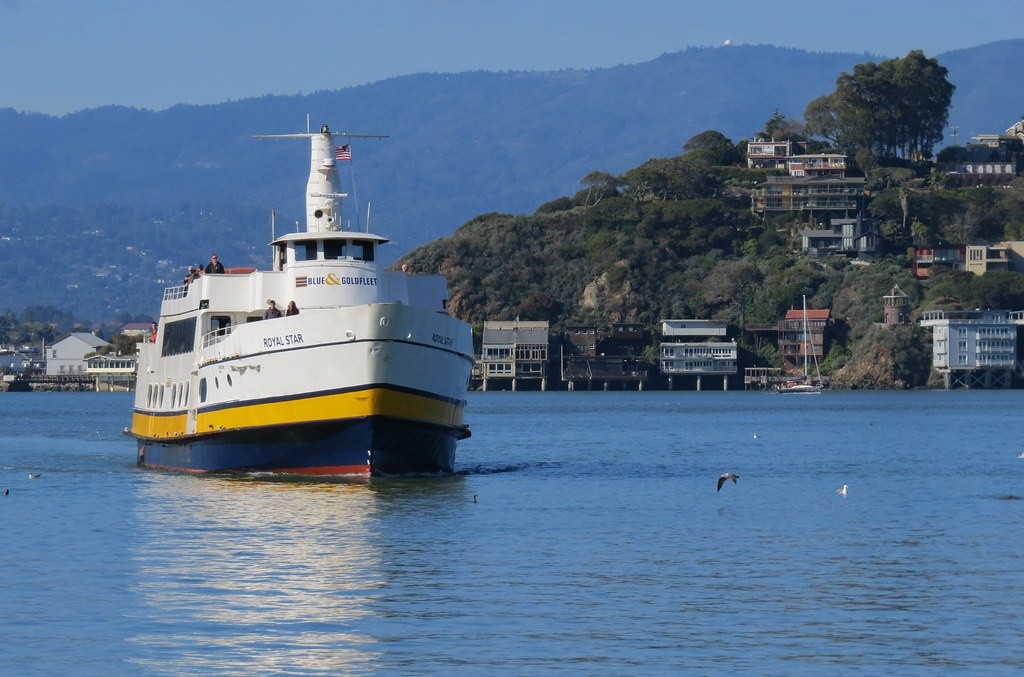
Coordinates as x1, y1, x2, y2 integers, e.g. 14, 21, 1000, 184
122, 113, 476, 485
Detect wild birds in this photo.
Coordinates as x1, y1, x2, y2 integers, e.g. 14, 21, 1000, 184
752, 432, 761, 441
717, 473, 743, 493
2, 471, 42, 497
834, 484, 850, 498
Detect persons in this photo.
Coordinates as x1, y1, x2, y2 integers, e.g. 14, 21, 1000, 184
182, 263, 203, 297
285, 301, 299, 316
263, 299, 282, 320
204, 254, 225, 274
149, 322, 157, 343
401, 263, 408, 272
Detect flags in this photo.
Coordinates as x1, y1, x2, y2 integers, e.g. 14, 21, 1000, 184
335, 143, 351, 160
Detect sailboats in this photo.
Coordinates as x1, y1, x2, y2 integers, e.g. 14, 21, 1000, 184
777, 294, 824, 393
